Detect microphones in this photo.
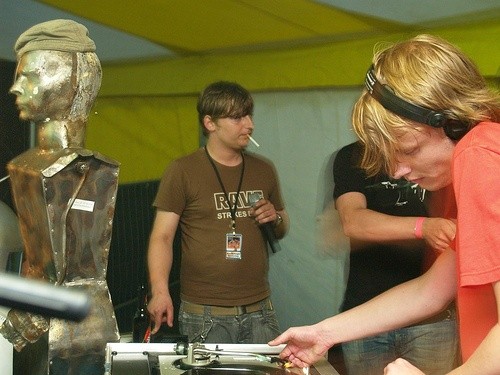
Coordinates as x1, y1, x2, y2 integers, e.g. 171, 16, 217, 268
250, 193, 282, 253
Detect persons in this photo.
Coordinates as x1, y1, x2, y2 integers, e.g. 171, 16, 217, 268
0, 19, 120, 352
147, 80, 290, 343
334, 139, 461, 375
268, 35, 500, 375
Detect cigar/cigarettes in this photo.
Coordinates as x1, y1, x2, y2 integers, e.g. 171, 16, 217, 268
246, 133, 260, 148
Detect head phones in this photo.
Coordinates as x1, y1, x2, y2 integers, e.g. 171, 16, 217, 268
362, 64, 474, 142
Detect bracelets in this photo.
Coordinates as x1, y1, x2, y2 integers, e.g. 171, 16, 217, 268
416, 217, 425, 240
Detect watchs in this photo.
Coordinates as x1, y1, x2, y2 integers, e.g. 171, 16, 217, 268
273, 213, 283, 227
